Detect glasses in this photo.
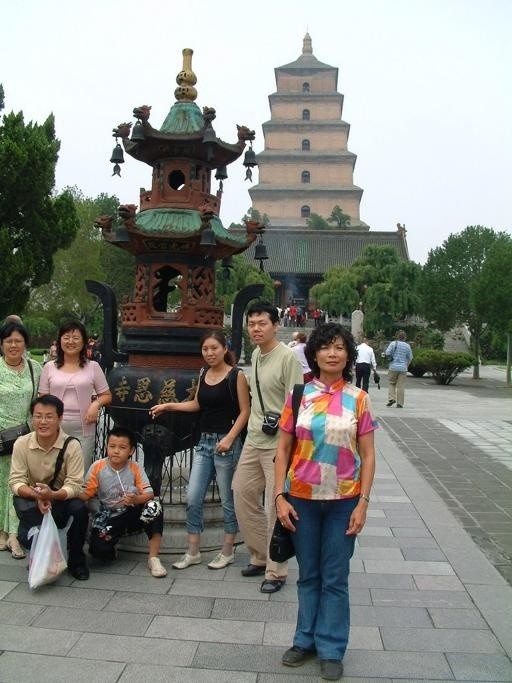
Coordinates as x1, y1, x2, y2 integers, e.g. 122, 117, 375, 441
31, 414, 55, 422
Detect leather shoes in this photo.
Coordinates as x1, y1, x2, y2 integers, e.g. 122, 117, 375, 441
241, 563, 266, 577
281, 644, 317, 667
68, 560, 90, 580
319, 658, 343, 681
260, 578, 282, 593
386, 399, 396, 407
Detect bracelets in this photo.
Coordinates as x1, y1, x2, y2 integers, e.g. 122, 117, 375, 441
358, 493, 373, 502
272, 492, 286, 507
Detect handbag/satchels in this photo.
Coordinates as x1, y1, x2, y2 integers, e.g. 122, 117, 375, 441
373, 372, 382, 390
267, 382, 306, 563
13, 436, 82, 521
386, 340, 398, 362
0, 359, 35, 456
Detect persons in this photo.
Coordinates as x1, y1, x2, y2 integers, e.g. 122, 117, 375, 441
147, 329, 250, 571
8, 393, 90, 582
49, 339, 57, 360
355, 338, 378, 394
90, 333, 100, 343
291, 332, 315, 385
77, 428, 167, 578
231, 298, 304, 591
1, 317, 44, 560
275, 301, 325, 328
380, 330, 413, 409
39, 320, 113, 478
275, 324, 380, 683
287, 331, 299, 347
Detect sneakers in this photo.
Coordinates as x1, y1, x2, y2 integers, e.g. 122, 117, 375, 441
172, 552, 203, 570
207, 552, 235, 569
147, 557, 167, 578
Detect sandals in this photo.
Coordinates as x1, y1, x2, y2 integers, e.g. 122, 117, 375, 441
6, 536, 26, 559
0, 530, 9, 551
